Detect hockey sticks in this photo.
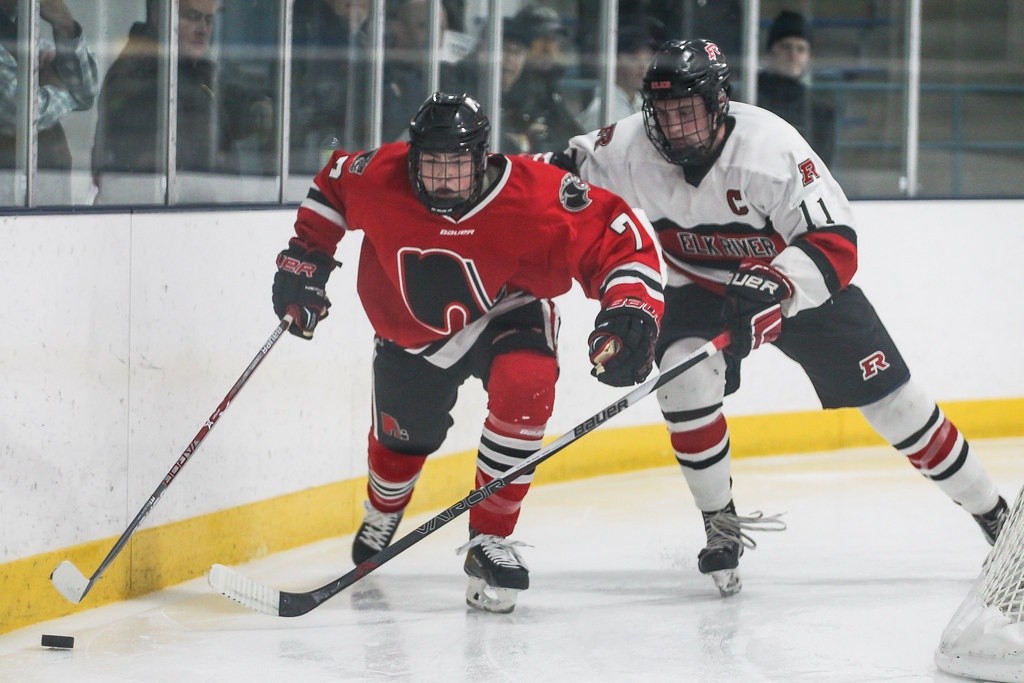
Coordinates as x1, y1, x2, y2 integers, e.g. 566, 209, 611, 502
206, 328, 730, 619
48, 308, 295, 606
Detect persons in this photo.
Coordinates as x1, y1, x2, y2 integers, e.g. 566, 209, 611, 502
518, 37, 1024, 599
760, 12, 834, 165
291, 0, 659, 155
0, 0, 278, 176
272, 92, 665, 613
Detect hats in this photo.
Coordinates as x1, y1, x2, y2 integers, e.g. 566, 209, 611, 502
767, 8, 813, 53
515, 5, 568, 39
487, 15, 530, 48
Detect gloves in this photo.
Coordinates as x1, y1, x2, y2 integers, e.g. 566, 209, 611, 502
517, 152, 576, 174
272, 243, 341, 339
587, 298, 661, 386
721, 256, 795, 360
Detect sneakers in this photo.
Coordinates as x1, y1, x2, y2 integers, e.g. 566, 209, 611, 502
453, 524, 536, 614
351, 501, 403, 566
698, 476, 786, 596
953, 494, 1011, 546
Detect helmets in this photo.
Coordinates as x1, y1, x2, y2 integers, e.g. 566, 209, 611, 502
640, 37, 730, 168
407, 90, 491, 214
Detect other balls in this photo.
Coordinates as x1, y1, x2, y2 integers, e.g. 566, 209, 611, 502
40, 633, 76, 649
